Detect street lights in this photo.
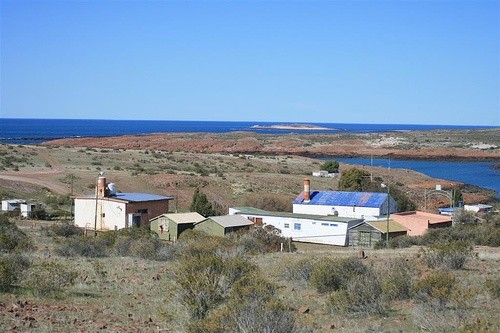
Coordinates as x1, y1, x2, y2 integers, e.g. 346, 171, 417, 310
93, 171, 106, 237
381, 182, 390, 246
